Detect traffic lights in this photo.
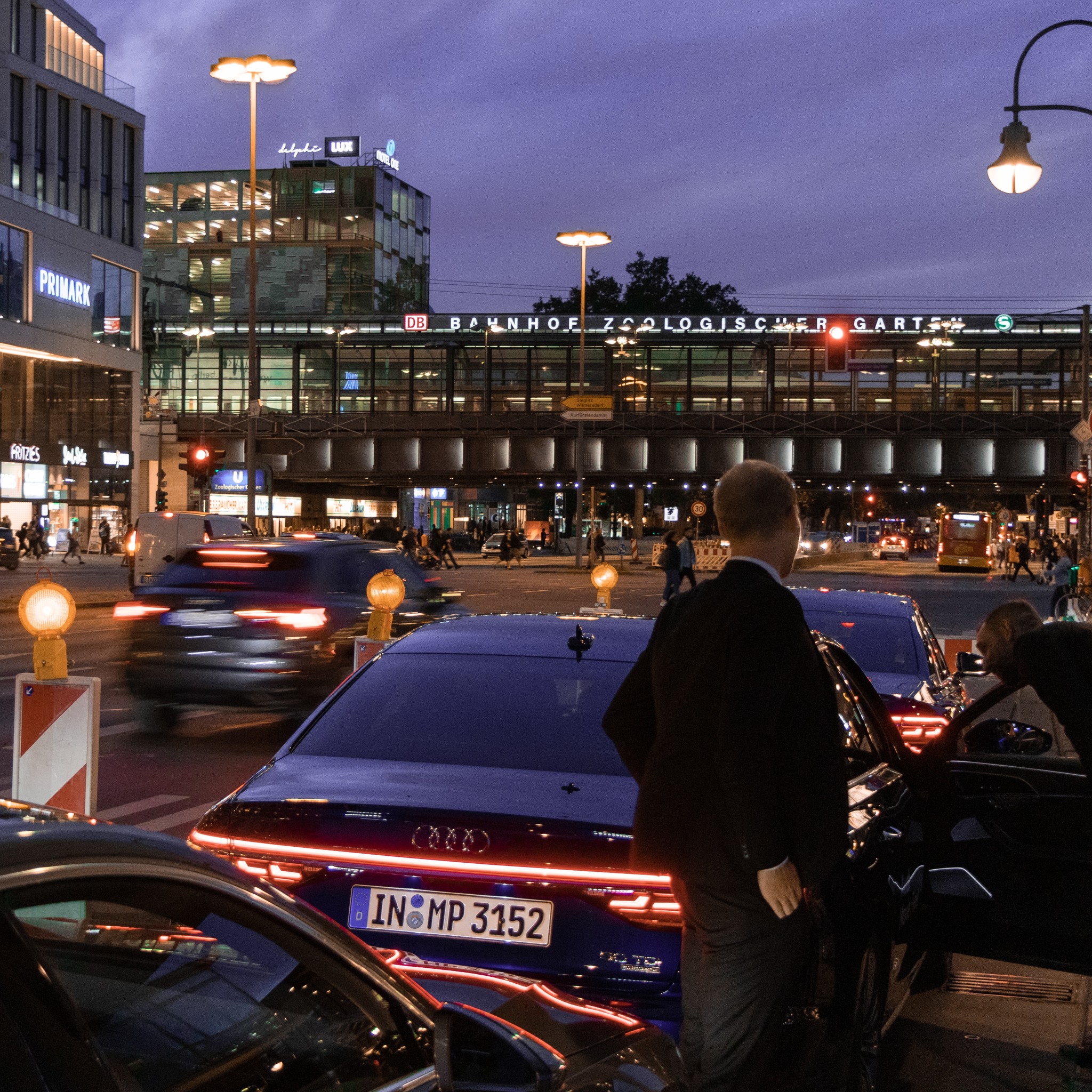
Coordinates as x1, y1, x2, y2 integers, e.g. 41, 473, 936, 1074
178, 447, 226, 489
1069, 471, 1086, 512
867, 495, 874, 503
865, 511, 872, 521
155, 491, 168, 512
826, 320, 847, 374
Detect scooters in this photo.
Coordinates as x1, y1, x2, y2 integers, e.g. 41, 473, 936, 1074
1037, 553, 1051, 585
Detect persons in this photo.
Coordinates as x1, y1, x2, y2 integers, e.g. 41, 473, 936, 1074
659, 529, 681, 606
601, 459, 849, 1092
1040, 543, 1072, 624
257, 517, 462, 570
62, 522, 87, 564
976, 599, 1092, 791
99, 516, 114, 555
991, 531, 1077, 586
0, 514, 51, 560
586, 527, 605, 569
670, 527, 697, 599
540, 528, 550, 552
121, 518, 134, 567
468, 516, 526, 570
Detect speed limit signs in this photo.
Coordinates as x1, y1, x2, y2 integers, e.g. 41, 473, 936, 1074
691, 502, 706, 516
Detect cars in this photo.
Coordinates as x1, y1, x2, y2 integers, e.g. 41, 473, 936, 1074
706, 531, 730, 547
801, 532, 852, 555
126, 530, 471, 714
0, 797, 683, 1090
878, 532, 938, 560
480, 530, 529, 558
188, 586, 1091, 1089
0, 527, 18, 570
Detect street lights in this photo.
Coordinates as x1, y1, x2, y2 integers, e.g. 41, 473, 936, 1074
323, 326, 357, 414
204, 54, 292, 536
558, 232, 613, 568
909, 319, 965, 412
181, 328, 215, 434
603, 321, 653, 412
771, 322, 809, 411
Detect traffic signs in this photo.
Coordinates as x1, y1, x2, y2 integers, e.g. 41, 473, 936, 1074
561, 396, 612, 421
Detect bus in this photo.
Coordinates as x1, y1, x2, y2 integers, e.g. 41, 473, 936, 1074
936, 511, 991, 573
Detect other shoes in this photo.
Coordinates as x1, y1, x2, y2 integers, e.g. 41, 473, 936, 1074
62, 560, 67, 564
455, 565, 461, 569
79, 561, 86, 564
446, 564, 452, 569
660, 601, 668, 606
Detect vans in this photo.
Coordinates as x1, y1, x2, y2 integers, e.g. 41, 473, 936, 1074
134, 511, 256, 586
